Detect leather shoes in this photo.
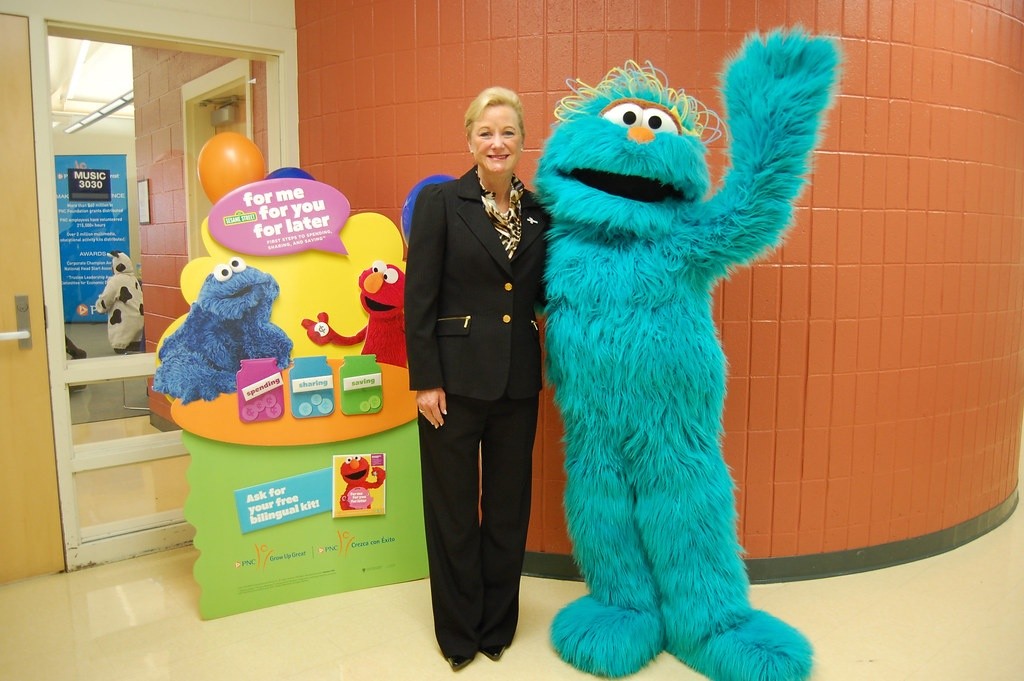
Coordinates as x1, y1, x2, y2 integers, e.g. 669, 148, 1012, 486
447, 656, 471, 670
481, 646, 506, 659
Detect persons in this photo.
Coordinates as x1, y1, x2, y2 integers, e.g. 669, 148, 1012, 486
65, 334, 87, 360
401, 88, 554, 671
96, 250, 145, 354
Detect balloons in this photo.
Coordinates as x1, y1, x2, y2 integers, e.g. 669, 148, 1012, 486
198, 131, 266, 207
268, 166, 316, 180
402, 174, 456, 248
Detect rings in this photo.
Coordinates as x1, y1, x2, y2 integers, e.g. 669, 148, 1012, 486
419, 410, 425, 413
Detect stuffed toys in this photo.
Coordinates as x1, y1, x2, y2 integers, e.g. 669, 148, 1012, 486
532, 25, 842, 681
301, 261, 406, 369
152, 257, 294, 407
339, 457, 386, 511
